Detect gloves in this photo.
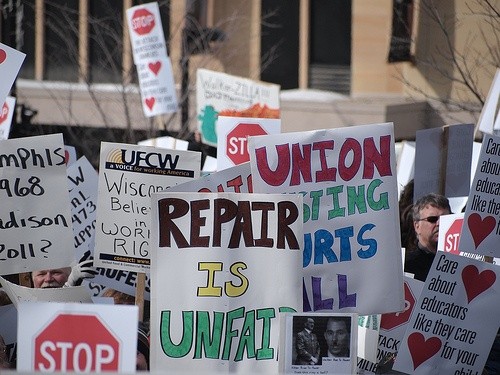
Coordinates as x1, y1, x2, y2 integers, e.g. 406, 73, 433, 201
66, 250, 100, 286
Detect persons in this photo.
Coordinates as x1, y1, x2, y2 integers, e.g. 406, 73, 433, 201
0, 177, 500, 375
297, 318, 320, 364
323, 316, 350, 358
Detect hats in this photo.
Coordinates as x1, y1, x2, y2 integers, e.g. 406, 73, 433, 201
137, 326, 149, 356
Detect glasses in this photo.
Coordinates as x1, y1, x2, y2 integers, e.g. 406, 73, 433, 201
417, 215, 440, 223
